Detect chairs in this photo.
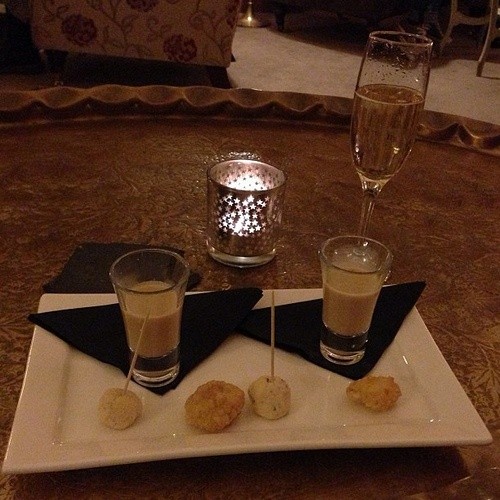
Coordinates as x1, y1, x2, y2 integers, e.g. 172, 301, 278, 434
438, 0, 500, 76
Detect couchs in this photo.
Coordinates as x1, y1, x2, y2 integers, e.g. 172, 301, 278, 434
5, 0, 240, 89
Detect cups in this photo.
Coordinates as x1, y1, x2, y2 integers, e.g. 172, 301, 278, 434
318, 235, 395, 367
108, 248, 191, 389
204, 159, 287, 268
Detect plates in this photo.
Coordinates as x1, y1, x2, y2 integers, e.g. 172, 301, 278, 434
0, 284, 495, 477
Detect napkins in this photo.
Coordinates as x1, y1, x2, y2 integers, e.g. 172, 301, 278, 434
43, 242, 202, 292
237, 282, 426, 380
28, 288, 263, 397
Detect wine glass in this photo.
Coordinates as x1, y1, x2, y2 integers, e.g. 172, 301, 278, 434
348, 30, 434, 235
